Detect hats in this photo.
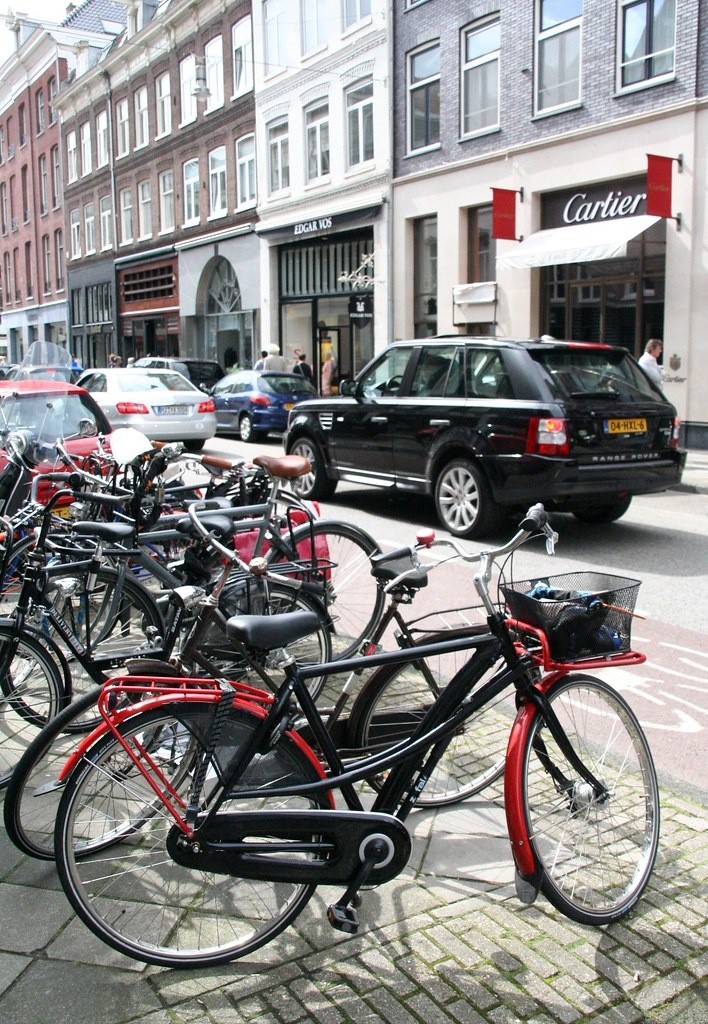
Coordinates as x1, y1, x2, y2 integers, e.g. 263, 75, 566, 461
269, 344, 280, 354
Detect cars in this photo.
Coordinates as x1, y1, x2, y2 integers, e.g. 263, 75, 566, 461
200, 369, 317, 443
0, 364, 86, 386
0, 380, 116, 509
52, 368, 218, 453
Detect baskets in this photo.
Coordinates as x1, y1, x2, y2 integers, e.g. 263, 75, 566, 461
499, 570, 643, 664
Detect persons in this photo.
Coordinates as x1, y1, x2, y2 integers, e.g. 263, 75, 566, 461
69, 354, 82, 377
293, 348, 302, 365
263, 344, 287, 373
638, 339, 669, 401
254, 351, 268, 370
0, 356, 8, 365
321, 352, 333, 396
293, 353, 311, 384
108, 354, 135, 368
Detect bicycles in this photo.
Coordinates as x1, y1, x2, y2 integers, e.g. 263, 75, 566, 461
52, 502, 663, 970
0, 340, 517, 861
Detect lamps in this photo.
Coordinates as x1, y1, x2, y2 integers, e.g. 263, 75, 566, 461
191, 60, 211, 103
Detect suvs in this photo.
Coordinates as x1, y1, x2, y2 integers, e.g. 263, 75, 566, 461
283, 333, 688, 541
132, 356, 224, 394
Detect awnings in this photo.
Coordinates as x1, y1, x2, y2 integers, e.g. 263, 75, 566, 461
496, 215, 666, 270
